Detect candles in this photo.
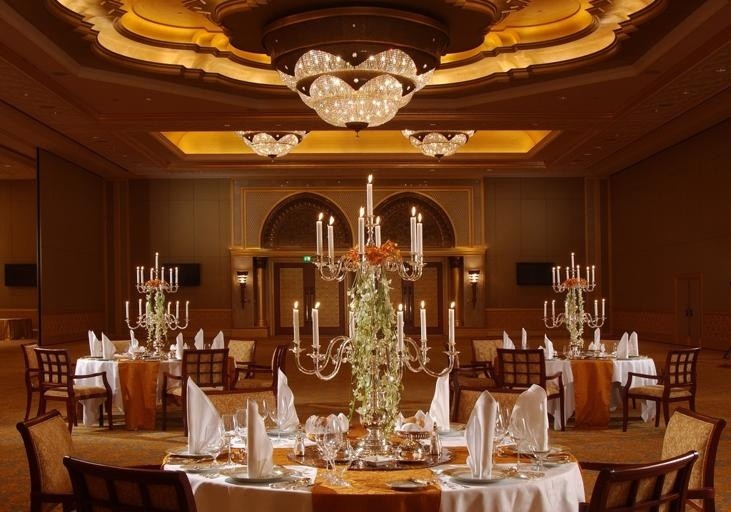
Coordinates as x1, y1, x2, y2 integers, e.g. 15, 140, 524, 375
552, 253, 595, 283
124, 298, 189, 322
292, 300, 457, 348
317, 174, 424, 260
544, 300, 605, 317
125, 253, 189, 292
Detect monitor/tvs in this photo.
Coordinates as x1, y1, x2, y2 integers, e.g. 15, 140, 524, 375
516, 263, 555, 285
162, 263, 200, 287
4, 264, 39, 287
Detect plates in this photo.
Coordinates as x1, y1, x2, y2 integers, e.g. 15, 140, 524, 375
231, 467, 291, 483
386, 480, 433, 492
441, 466, 510, 484
165, 445, 224, 458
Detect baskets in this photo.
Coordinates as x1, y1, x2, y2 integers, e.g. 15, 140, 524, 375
400, 431, 430, 440
308, 432, 347, 442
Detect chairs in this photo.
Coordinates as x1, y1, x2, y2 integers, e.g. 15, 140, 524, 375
579, 409, 724, 512
590, 449, 698, 512
64, 455, 196, 512
17, 409, 161, 512
20, 339, 288, 431
454, 337, 701, 435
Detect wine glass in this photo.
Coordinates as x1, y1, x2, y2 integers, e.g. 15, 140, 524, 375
207, 437, 224, 465
315, 415, 353, 487
269, 396, 289, 446
509, 417, 528, 468
233, 410, 251, 451
530, 436, 551, 471
251, 397, 267, 427
220, 413, 238, 466
496, 406, 510, 458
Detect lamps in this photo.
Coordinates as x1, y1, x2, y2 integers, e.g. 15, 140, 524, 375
401, 129, 475, 160
468, 269, 480, 308
237, 271, 249, 310
234, 130, 309, 159
55, 0, 659, 139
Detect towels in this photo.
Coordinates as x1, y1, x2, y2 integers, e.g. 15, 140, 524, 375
184, 366, 552, 482
501, 326, 640, 361
86, 327, 226, 361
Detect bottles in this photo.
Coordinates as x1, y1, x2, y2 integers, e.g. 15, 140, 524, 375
430, 422, 442, 455
295, 424, 305, 456
